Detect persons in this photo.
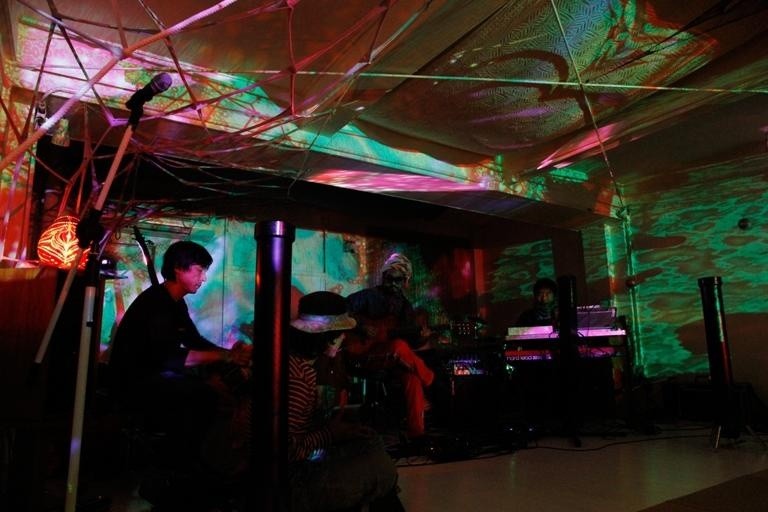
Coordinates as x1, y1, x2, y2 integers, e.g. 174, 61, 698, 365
224, 288, 401, 511
345, 253, 453, 457
99, 236, 254, 476
411, 303, 436, 351
516, 277, 564, 347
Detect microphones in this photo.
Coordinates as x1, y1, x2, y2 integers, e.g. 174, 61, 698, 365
125, 73, 172, 109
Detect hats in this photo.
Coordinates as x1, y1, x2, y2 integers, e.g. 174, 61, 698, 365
288, 290, 359, 333
381, 252, 413, 279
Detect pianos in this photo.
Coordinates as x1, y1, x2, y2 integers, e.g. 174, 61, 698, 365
503, 327, 627, 362
